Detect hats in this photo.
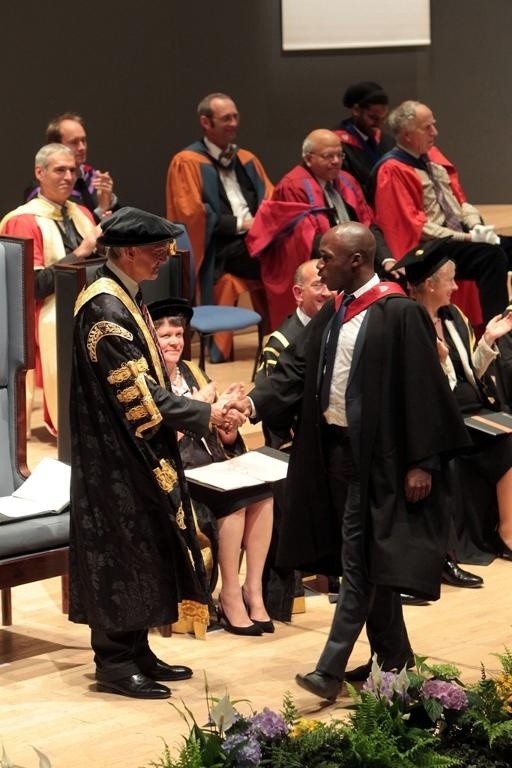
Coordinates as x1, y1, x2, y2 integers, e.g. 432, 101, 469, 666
147, 296, 193, 322
388, 235, 456, 286
96, 206, 185, 248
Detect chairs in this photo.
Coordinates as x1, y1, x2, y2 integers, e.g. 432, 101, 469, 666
0, 388, 73, 626
173, 223, 261, 380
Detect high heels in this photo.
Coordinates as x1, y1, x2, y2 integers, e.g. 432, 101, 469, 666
493, 524, 512, 560
216, 590, 276, 634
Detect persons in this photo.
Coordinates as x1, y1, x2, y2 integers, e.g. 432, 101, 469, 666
386, 233, 512, 563
166, 91, 275, 364
25, 111, 120, 226
0, 142, 112, 439
68, 203, 247, 702
254, 259, 483, 588
245, 127, 406, 334
367, 99, 511, 326
142, 294, 276, 637
330, 79, 396, 195
218, 220, 474, 704
496, 297, 512, 410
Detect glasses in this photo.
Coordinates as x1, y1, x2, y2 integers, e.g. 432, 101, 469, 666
215, 112, 239, 121
310, 151, 346, 160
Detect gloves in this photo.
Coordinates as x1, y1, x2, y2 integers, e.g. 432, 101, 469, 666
470, 223, 502, 245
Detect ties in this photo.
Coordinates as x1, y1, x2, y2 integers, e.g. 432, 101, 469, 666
422, 154, 464, 232
319, 294, 355, 411
61, 206, 78, 248
325, 181, 350, 224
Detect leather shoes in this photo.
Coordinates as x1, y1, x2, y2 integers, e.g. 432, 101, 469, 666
400, 593, 428, 605
440, 556, 483, 588
